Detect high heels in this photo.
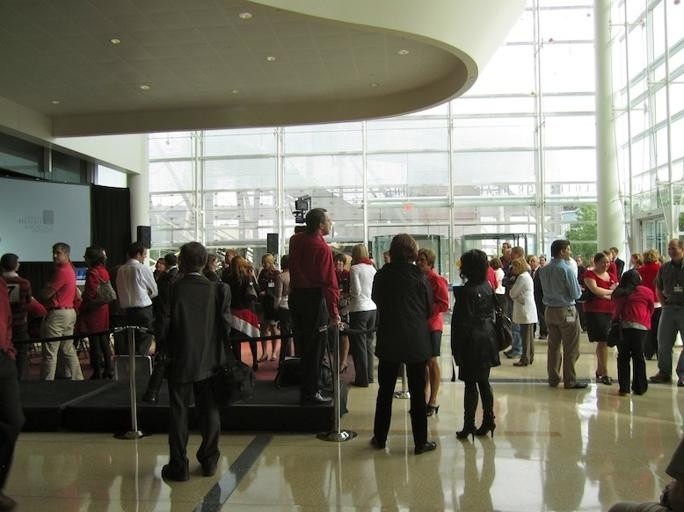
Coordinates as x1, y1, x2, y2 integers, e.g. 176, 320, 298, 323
513, 362, 533, 366
426, 403, 439, 417
455, 411, 496, 441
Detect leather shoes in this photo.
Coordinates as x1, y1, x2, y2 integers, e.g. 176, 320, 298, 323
415, 442, 437, 454
572, 383, 588, 389
596, 372, 613, 385
302, 389, 333, 405
257, 354, 276, 362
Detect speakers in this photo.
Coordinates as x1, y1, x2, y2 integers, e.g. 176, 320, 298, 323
267, 233, 278, 255
137, 226, 151, 250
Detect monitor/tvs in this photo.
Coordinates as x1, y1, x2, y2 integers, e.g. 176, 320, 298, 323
76, 267, 89, 280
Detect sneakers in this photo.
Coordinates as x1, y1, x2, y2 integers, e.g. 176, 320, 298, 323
649, 372, 671, 383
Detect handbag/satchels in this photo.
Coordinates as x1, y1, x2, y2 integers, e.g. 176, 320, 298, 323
245, 284, 258, 302
576, 286, 593, 305
279, 356, 334, 392
609, 319, 624, 345
493, 312, 512, 351
81, 280, 118, 309
213, 360, 256, 406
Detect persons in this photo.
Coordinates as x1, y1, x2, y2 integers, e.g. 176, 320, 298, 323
369, 233, 450, 455
611, 443, 682, 512
538, 239, 588, 388
288, 208, 377, 405
1, 254, 33, 510
451, 249, 496, 441
34, 240, 159, 383
581, 239, 684, 395
486, 242, 548, 367
149, 242, 290, 482
